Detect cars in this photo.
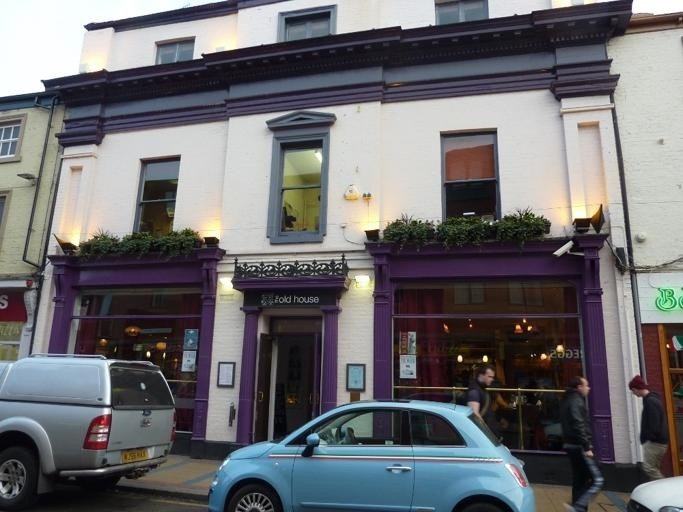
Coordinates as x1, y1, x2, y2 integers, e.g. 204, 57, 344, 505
627, 476, 683, 512
167, 377, 197, 426
207, 399, 535, 512
406, 391, 513, 442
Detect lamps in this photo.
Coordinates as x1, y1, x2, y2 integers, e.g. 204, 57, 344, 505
363, 229, 381, 242
572, 218, 592, 234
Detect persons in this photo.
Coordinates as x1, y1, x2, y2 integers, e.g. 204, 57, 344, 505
466, 364, 507, 432
560, 376, 605, 512
629, 375, 669, 481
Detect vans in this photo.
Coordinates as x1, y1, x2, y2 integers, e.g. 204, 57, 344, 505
0, 354, 178, 509
